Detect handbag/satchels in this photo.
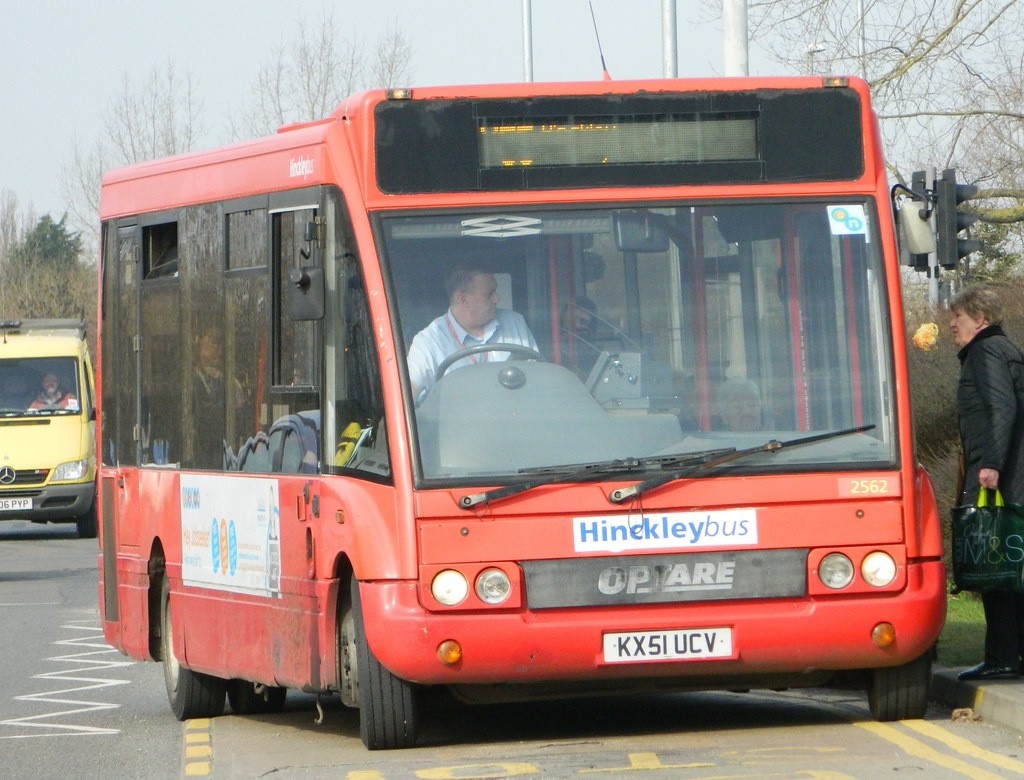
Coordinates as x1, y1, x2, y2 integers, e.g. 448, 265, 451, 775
950, 482, 1024, 597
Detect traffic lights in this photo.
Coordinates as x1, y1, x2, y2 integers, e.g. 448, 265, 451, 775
938, 168, 981, 270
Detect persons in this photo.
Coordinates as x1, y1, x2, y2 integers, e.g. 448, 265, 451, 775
949, 284, 1024, 681
560, 297, 597, 340
194, 322, 243, 394
407, 261, 539, 409
27, 371, 76, 410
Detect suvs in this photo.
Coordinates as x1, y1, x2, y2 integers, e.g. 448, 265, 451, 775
0, 303, 96, 537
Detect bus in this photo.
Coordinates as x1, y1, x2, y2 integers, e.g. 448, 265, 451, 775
95, 75, 949, 752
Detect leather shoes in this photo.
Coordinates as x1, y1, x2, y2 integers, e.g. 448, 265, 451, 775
956, 659, 1024, 681
